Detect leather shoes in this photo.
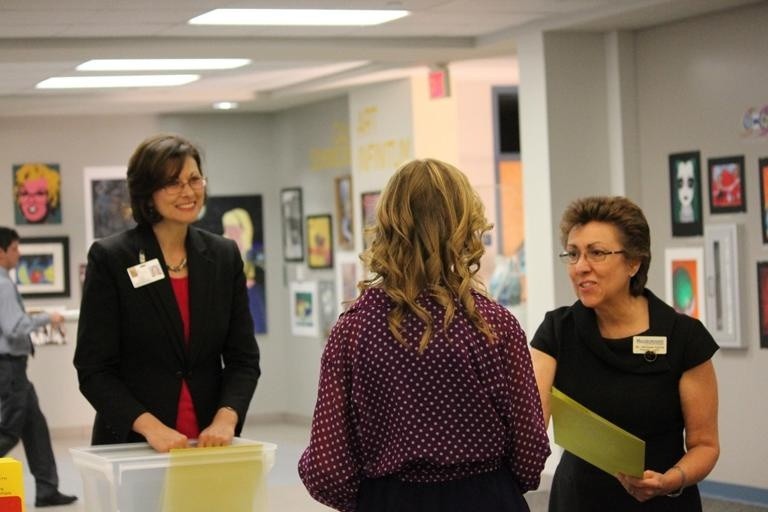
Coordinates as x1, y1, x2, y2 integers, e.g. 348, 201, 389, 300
34, 490, 79, 507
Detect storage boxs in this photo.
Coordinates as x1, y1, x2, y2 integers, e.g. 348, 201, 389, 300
65, 431, 276, 510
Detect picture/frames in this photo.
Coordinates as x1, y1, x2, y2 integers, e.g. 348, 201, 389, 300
361, 190, 382, 253
662, 242, 705, 322
754, 258, 766, 349
705, 154, 750, 216
698, 220, 746, 355
10, 233, 73, 302
278, 186, 307, 265
305, 214, 336, 272
332, 175, 355, 251
757, 149, 768, 244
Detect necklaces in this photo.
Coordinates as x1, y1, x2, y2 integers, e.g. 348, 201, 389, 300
166, 256, 188, 273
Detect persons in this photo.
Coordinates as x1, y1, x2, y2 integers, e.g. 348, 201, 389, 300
528, 194, 720, 512
296, 157, 550, 511
0, 226, 78, 507
72, 134, 260, 453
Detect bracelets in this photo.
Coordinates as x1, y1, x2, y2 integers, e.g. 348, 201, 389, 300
666, 466, 685, 499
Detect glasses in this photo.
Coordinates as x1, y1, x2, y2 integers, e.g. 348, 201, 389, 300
161, 173, 207, 195
558, 245, 626, 265
